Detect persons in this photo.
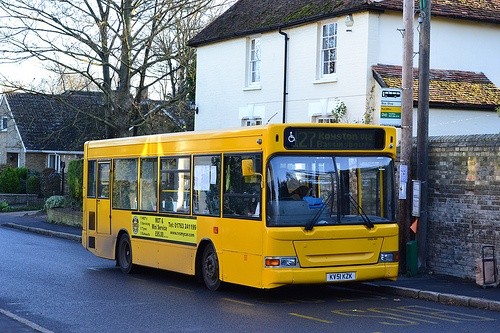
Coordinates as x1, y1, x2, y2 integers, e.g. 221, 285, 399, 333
291, 186, 313, 201
101, 181, 259, 216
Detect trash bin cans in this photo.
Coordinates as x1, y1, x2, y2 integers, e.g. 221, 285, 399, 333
405, 240, 419, 278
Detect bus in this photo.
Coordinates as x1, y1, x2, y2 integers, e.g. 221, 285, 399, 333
83, 122, 399, 297
